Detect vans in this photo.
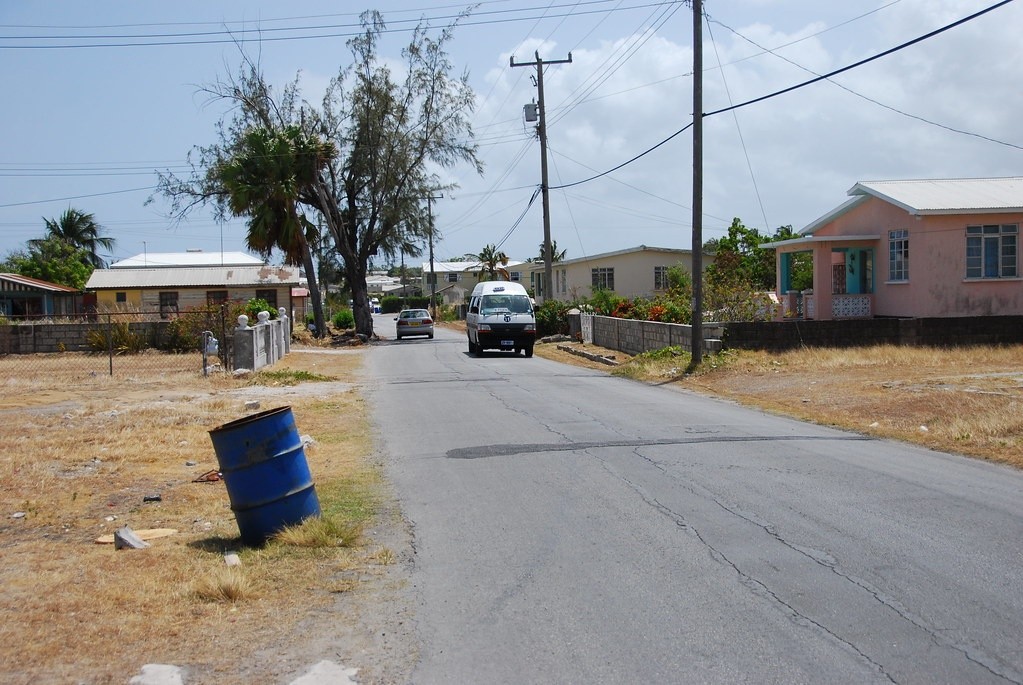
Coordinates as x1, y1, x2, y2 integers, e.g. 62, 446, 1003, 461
465, 281, 538, 358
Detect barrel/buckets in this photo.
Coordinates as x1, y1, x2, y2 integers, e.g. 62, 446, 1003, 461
207, 404, 322, 548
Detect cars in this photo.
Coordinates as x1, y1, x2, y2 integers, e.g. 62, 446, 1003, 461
394, 309, 434, 339
